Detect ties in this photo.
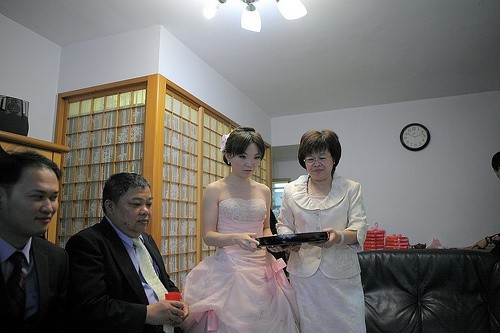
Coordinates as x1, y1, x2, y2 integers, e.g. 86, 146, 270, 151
132, 237, 174, 333
6, 252, 27, 324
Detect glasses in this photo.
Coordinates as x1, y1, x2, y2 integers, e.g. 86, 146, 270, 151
304, 153, 332, 163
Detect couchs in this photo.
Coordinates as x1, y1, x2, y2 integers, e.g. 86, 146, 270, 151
358, 247, 500, 333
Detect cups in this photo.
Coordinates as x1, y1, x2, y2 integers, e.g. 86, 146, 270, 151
165, 292, 181, 301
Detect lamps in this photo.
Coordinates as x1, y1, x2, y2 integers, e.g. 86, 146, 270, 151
202, 0, 308, 33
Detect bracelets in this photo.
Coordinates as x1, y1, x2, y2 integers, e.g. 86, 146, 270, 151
486, 236, 492, 245
336, 230, 345, 245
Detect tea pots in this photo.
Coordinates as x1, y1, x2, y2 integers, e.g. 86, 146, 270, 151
411, 243, 426, 249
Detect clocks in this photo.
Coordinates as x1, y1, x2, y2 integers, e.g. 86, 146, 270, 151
400, 123, 431, 152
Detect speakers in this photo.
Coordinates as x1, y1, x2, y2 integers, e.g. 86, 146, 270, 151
0, 95, 29, 136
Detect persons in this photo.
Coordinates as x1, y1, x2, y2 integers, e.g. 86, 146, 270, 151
65, 173, 190, 332
1, 150, 71, 332
180, 125, 299, 332
468, 150, 500, 261
274, 129, 371, 333
